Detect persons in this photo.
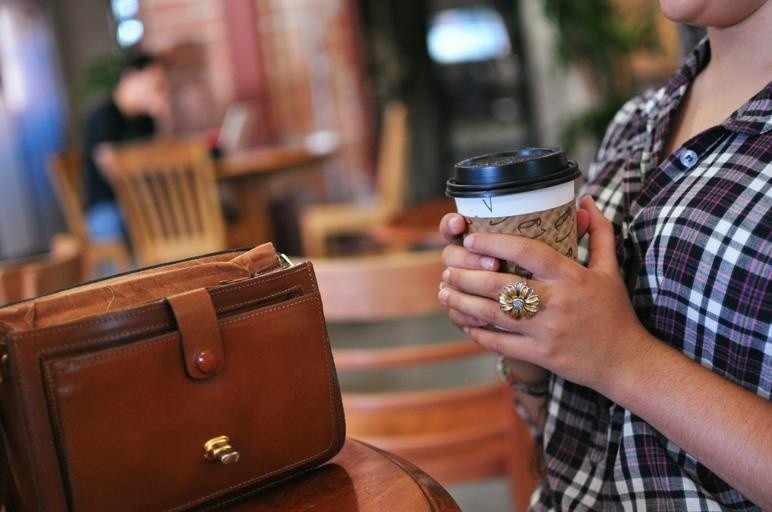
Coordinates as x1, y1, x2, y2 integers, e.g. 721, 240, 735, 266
80, 51, 177, 275
436, 0, 772, 512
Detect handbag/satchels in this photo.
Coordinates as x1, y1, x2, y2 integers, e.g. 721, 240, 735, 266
0, 242, 346, 511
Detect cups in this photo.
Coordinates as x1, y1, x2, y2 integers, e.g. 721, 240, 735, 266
443, 145, 583, 334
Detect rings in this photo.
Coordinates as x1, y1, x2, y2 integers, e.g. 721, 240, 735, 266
497, 275, 541, 321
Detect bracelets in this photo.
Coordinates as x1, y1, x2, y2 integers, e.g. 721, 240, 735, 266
492, 354, 551, 398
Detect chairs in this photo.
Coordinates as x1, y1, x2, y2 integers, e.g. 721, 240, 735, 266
43, 137, 226, 282
299, 104, 407, 258
282, 250, 548, 512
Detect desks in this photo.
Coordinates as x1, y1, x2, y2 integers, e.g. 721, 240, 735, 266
214, 128, 345, 250
